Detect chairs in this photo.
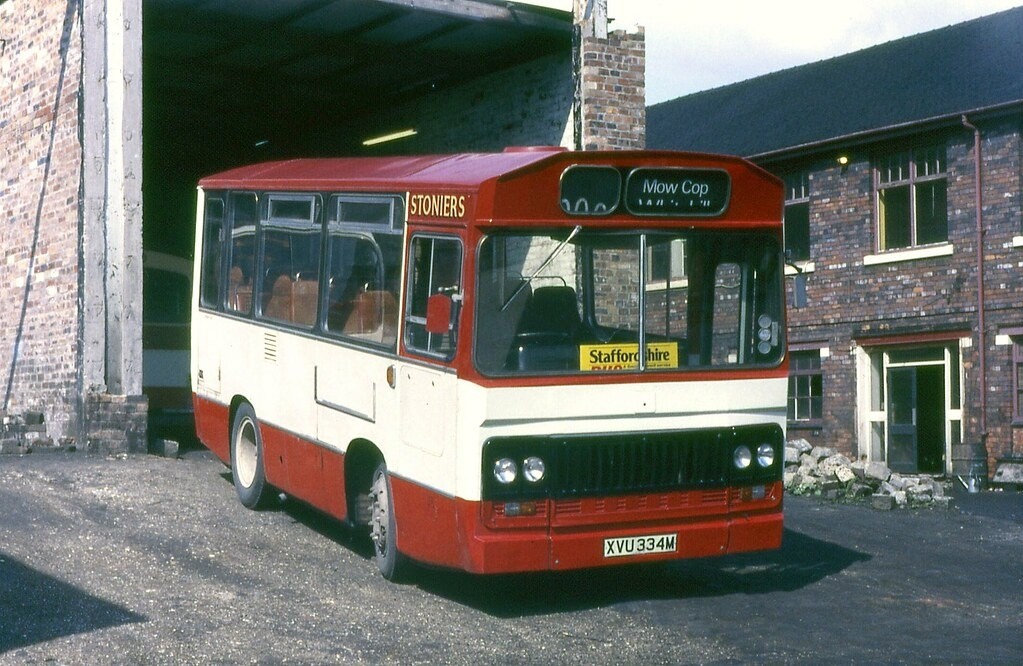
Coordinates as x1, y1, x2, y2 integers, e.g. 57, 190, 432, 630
229, 265, 398, 347
534, 284, 599, 344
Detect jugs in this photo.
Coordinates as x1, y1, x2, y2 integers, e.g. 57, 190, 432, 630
957, 469, 982, 494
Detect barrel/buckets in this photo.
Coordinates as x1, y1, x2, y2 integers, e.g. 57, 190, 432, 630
950, 441, 991, 495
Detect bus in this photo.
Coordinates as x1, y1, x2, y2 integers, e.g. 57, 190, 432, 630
190, 146, 807, 583
